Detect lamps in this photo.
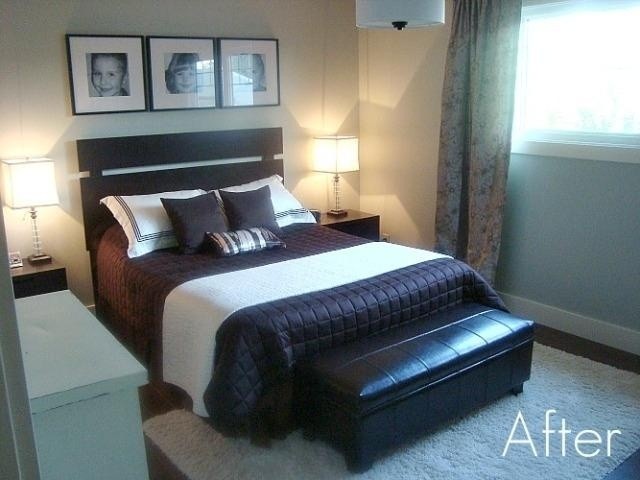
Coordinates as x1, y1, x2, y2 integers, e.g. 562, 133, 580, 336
311, 136, 360, 218
355, 0, 445, 31
0, 156, 59, 265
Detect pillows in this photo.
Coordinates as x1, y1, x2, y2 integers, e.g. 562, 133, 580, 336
206, 230, 287, 254
101, 189, 210, 260
219, 186, 286, 237
217, 175, 316, 230
160, 192, 230, 254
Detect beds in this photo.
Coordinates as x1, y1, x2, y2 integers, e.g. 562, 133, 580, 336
76, 127, 510, 448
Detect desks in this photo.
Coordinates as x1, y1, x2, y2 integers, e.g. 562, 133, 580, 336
13, 290, 150, 480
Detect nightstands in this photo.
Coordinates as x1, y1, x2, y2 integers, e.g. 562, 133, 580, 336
316, 209, 379, 242
10, 255, 68, 298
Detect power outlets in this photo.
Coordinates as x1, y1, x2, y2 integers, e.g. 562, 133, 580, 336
381, 233, 389, 243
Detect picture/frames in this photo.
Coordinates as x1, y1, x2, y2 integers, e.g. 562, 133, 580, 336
65, 31, 150, 115
144, 34, 220, 112
215, 37, 282, 110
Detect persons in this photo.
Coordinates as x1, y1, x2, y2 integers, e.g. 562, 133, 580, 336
91, 53, 129, 97
236, 54, 268, 91
165, 53, 206, 94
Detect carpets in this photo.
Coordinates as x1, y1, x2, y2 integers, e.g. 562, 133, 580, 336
142, 341, 640, 480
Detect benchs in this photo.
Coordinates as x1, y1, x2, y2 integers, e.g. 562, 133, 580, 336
297, 301, 536, 473
